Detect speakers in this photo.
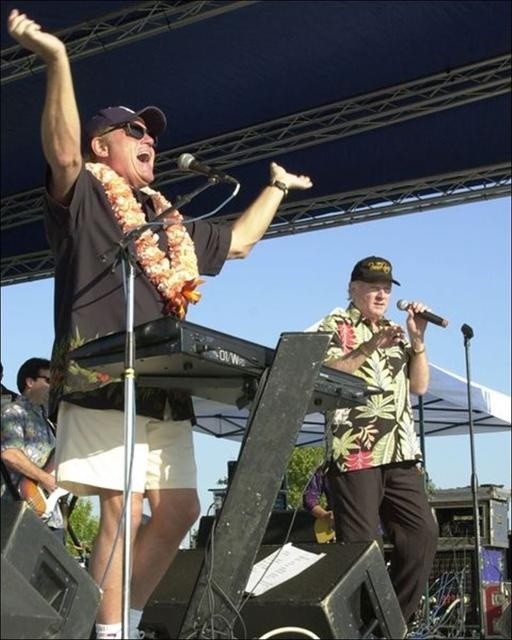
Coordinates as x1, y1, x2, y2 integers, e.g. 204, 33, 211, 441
138, 539, 409, 640
1, 498, 106, 640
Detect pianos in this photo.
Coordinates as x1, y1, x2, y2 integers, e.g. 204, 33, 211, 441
69, 315, 372, 413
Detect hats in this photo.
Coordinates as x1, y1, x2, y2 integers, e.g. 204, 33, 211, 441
83, 104, 168, 137
349, 256, 401, 288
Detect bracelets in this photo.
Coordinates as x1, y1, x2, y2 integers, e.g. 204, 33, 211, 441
267, 180, 289, 197
410, 344, 425, 354
359, 343, 372, 358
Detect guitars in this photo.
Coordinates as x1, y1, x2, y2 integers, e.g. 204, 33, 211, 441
15, 448, 81, 532
315, 514, 338, 545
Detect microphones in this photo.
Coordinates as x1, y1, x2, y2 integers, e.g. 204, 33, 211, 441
176, 150, 238, 187
395, 299, 449, 329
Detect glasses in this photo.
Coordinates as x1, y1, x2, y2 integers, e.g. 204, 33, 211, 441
97, 121, 160, 147
31, 374, 51, 384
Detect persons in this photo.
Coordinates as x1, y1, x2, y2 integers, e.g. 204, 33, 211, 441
0, 361, 20, 413
1, 359, 73, 547
302, 461, 337, 544
318, 257, 440, 639
6, 7, 312, 640
